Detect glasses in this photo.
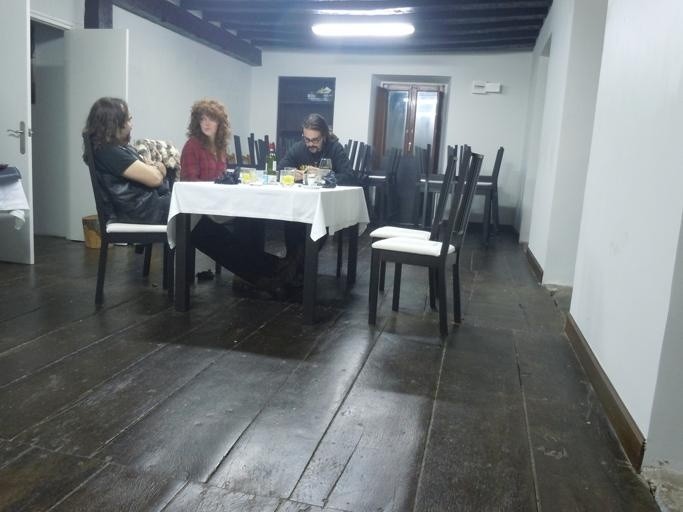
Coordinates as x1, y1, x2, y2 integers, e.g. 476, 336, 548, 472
302, 135, 322, 144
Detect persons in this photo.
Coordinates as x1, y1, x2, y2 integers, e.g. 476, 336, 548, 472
276, 113, 354, 259
181, 99, 266, 287
82, 97, 303, 299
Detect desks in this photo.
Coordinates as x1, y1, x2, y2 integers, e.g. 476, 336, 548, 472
166, 180, 370, 327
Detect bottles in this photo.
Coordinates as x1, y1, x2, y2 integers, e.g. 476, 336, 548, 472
264, 149, 278, 175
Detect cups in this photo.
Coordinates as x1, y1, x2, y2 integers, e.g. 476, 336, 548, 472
279, 168, 296, 186
317, 157, 332, 169
240, 166, 257, 185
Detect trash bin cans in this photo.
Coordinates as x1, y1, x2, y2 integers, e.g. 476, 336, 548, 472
82, 215, 114, 248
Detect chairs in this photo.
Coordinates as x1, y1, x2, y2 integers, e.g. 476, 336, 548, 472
367, 153, 484, 334
226, 135, 503, 291
83, 132, 176, 307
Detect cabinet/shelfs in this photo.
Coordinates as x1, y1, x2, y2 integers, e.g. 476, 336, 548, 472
276, 75, 336, 149
